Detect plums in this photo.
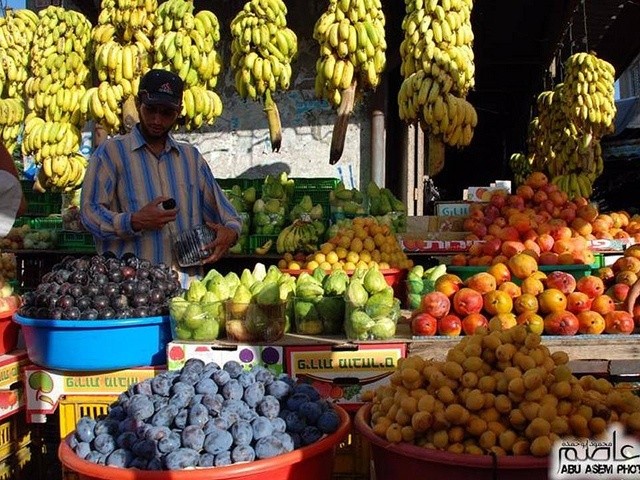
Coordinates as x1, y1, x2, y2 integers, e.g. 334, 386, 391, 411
20, 250, 180, 321
68, 358, 338, 471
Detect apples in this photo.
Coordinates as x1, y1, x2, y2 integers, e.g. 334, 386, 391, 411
422, 170, 640, 267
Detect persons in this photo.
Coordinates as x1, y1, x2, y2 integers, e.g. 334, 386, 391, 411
80, 69, 242, 275
0, 138, 28, 237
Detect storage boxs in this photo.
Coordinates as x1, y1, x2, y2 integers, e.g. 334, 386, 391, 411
1, 348, 30, 419
285, 344, 409, 411
165, 339, 285, 371
434, 199, 473, 218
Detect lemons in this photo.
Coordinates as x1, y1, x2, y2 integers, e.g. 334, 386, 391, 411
316, 218, 414, 270
278, 253, 316, 269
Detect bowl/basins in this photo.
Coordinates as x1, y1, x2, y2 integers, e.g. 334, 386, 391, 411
404, 280, 443, 310
355, 401, 619, 479
167, 301, 225, 342
446, 265, 591, 287
0, 311, 18, 354
288, 213, 324, 223
343, 297, 401, 341
331, 200, 372, 221
293, 296, 343, 335
280, 268, 409, 288
253, 211, 285, 235
57, 400, 352, 480
11, 311, 174, 372
386, 212, 406, 232
222, 299, 289, 344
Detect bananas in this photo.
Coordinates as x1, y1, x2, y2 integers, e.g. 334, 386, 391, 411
508, 52, 616, 200
312, 0, 387, 106
229, 0, 298, 102
0, 9, 39, 157
92, 0, 158, 133
158, 1, 223, 132
277, 219, 326, 253
21, 5, 91, 192
398, 0, 479, 151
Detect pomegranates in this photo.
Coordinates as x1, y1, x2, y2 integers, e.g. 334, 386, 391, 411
409, 245, 640, 336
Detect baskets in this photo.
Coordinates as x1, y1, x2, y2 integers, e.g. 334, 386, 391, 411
58, 393, 121, 478
